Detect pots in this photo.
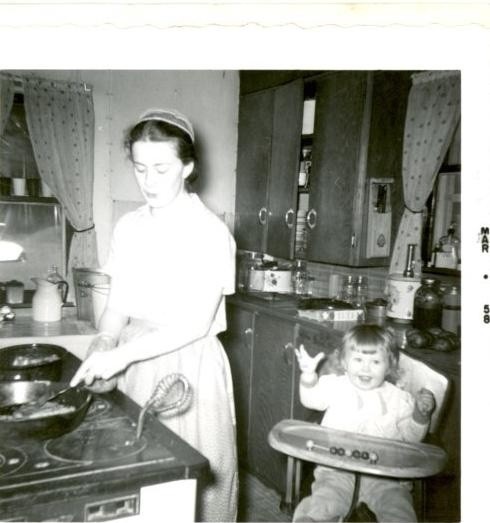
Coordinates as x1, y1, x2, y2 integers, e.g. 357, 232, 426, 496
1, 379, 92, 441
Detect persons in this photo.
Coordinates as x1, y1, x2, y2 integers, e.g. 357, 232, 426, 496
287, 324, 437, 523
70, 108, 239, 522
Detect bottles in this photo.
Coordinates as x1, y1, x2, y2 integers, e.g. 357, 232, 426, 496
352, 275, 368, 306
341, 275, 351, 302
238, 252, 253, 293
293, 259, 305, 295
439, 228, 460, 250
441, 286, 461, 339
251, 253, 266, 271
46, 265, 64, 300
412, 278, 442, 329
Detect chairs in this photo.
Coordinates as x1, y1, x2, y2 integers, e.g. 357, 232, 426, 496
269, 353, 450, 521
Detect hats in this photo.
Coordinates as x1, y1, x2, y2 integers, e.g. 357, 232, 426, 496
130, 108, 197, 146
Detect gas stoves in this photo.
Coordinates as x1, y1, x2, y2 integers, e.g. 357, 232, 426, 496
1, 341, 211, 513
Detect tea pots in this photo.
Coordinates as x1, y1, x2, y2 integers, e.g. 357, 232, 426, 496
31, 277, 69, 322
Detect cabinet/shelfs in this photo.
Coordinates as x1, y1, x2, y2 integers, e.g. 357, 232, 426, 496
2, 96, 69, 317
217, 299, 256, 478
263, 71, 424, 266
234, 89, 267, 252
251, 308, 351, 506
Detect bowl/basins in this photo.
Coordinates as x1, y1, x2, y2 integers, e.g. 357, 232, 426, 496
0, 342, 67, 382
